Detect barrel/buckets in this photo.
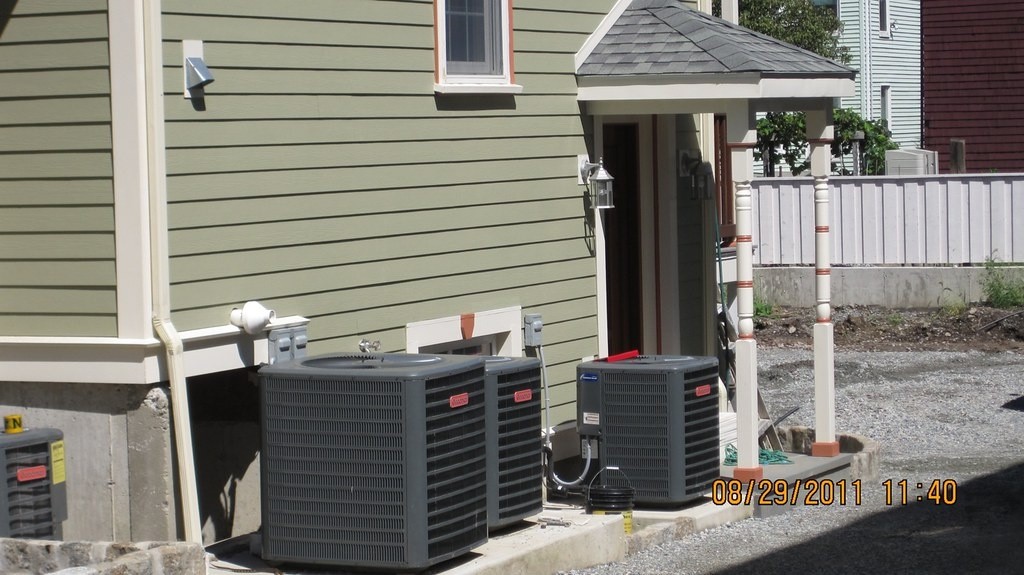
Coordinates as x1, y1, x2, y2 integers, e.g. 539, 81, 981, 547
585, 466, 635, 536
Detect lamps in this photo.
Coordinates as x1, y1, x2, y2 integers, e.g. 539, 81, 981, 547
578, 153, 615, 209
680, 148, 714, 199
